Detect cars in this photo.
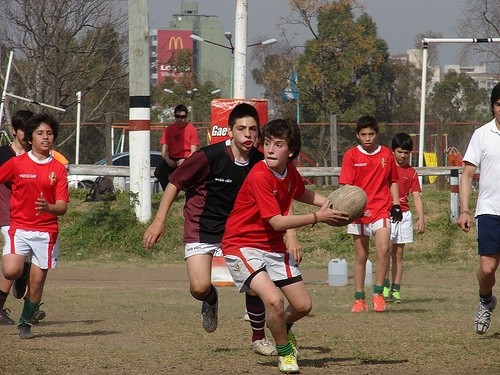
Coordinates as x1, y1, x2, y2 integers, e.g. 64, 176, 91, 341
66, 150, 181, 201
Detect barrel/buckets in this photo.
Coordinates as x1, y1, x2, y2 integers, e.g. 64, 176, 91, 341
328, 258, 348, 286
364, 259, 373, 286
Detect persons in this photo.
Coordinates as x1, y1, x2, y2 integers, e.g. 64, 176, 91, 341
222, 119, 349, 374
456, 82, 500, 335
154, 104, 200, 193
0, 110, 46, 326
142, 102, 278, 357
339, 115, 403, 312
0, 113, 70, 339
382, 132, 424, 304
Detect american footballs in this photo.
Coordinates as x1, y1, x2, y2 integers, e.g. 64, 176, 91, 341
326, 184, 368, 228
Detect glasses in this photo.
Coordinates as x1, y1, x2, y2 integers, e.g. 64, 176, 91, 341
175, 115, 187, 119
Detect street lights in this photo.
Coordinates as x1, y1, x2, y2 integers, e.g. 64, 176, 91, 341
190, 32, 278, 100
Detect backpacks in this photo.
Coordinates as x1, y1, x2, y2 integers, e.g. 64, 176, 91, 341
86, 177, 115, 202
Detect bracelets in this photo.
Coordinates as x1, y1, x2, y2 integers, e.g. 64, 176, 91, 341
458, 211, 470, 218
310, 213, 318, 228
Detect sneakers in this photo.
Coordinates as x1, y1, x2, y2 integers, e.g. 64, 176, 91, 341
200, 288, 219, 334
287, 328, 297, 351
372, 293, 387, 312
382, 285, 391, 303
13, 262, 29, 299
389, 290, 402, 304
17, 322, 34, 338
277, 351, 300, 373
0, 307, 15, 324
252, 337, 278, 356
474, 295, 497, 335
31, 307, 46, 326
351, 298, 368, 314
